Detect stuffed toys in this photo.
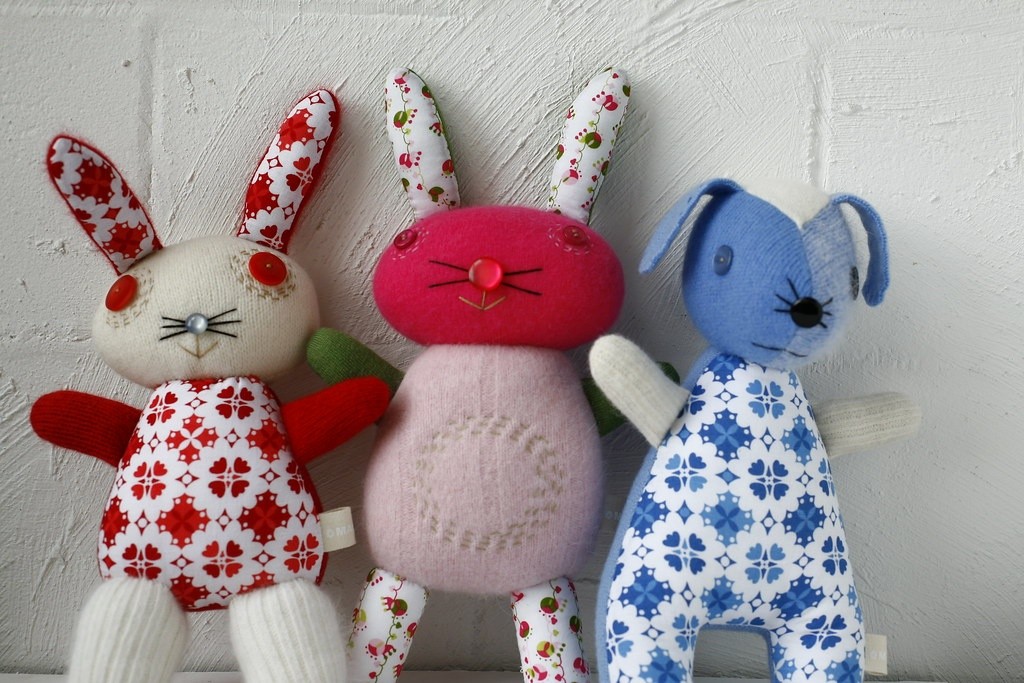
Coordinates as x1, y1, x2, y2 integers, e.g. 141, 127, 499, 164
298, 58, 636, 682
30, 87, 392, 683
587, 178, 899, 683
